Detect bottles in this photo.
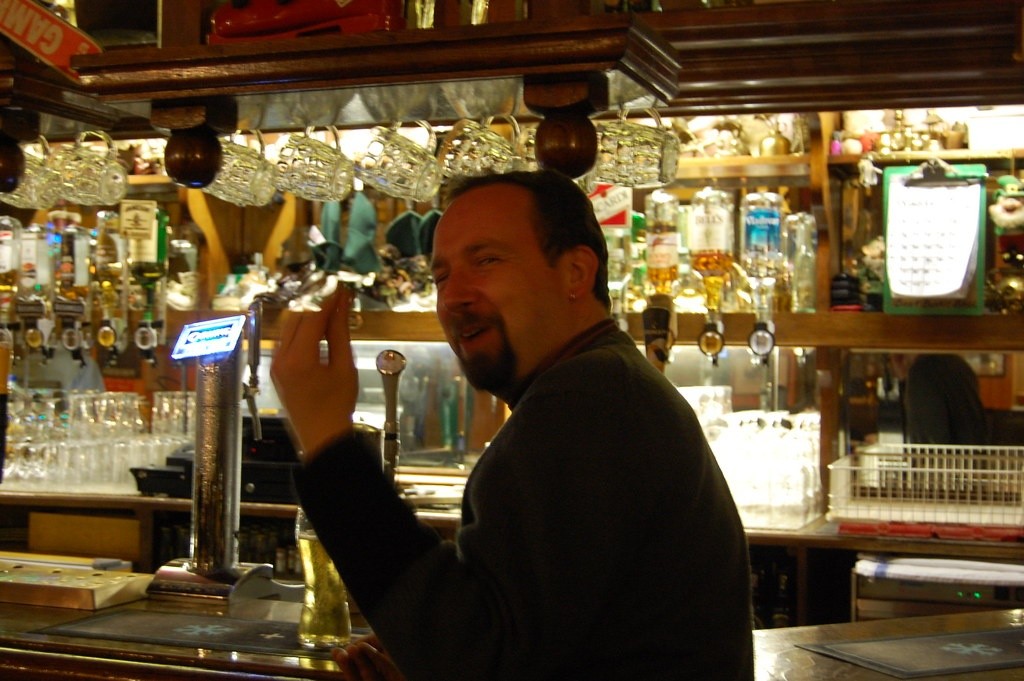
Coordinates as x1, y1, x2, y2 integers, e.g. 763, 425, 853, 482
790, 212, 816, 311
587, 181, 781, 334
0, 208, 168, 350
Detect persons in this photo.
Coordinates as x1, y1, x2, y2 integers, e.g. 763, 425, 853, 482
270, 169, 754, 680
904, 353, 990, 476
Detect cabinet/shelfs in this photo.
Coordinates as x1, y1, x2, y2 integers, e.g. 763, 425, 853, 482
0, 141, 1024, 358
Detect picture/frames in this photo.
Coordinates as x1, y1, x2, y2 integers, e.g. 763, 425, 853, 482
976, 355, 1006, 377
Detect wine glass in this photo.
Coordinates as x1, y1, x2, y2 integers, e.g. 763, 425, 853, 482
680, 386, 820, 531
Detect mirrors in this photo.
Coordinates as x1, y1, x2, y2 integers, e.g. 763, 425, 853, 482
4, 331, 1022, 471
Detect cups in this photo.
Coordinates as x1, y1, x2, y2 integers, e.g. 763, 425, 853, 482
354, 118, 442, 203
519, 130, 539, 170
592, 106, 681, 188
272, 124, 353, 203
295, 507, 352, 648
201, 128, 276, 207
0, 135, 62, 208
51, 130, 128, 206
3, 388, 196, 494
437, 115, 519, 179
